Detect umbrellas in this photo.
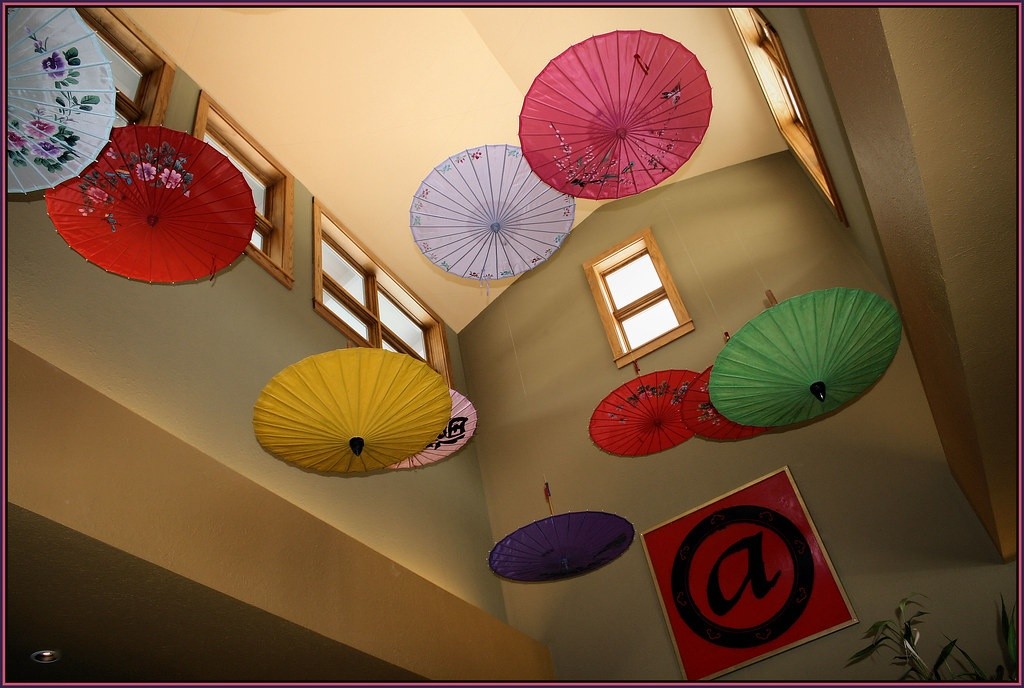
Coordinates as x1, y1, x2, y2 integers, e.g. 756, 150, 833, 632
8, 8, 120, 196
44, 122, 256, 284
589, 289, 901, 458
411, 144, 576, 282
519, 30, 713, 201
253, 340, 479, 473
486, 482, 635, 581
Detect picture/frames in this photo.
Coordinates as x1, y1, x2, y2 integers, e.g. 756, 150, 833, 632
638, 464, 860, 680
